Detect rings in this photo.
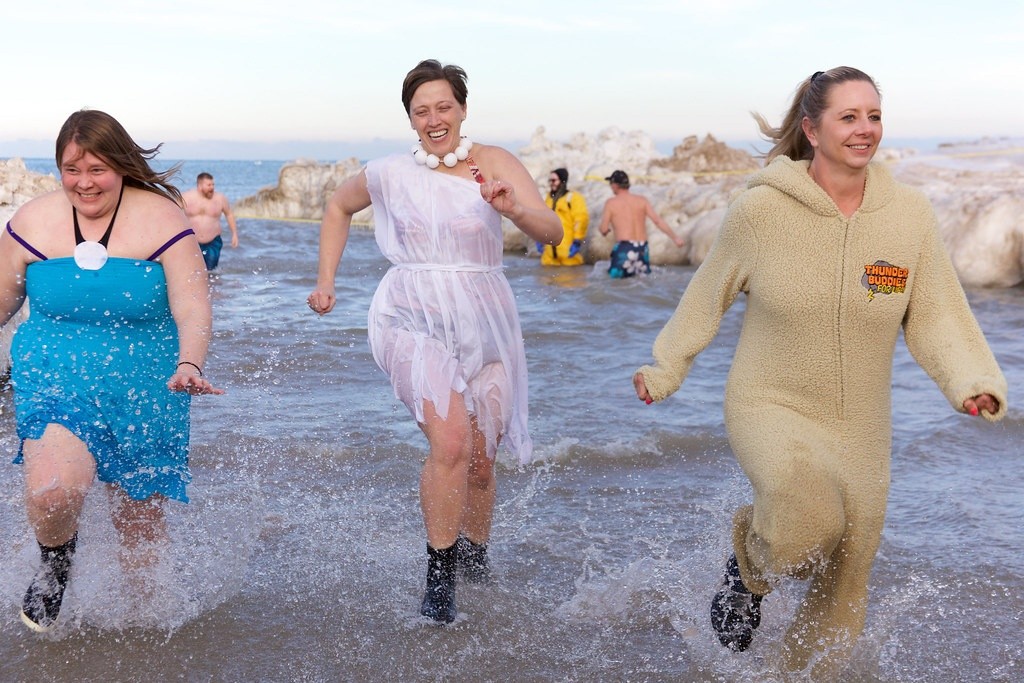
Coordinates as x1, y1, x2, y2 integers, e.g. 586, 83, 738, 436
497, 180, 502, 185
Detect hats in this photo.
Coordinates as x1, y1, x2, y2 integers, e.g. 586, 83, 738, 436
550, 168, 568, 183
605, 170, 629, 183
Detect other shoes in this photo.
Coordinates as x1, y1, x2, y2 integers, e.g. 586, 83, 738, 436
711, 553, 763, 654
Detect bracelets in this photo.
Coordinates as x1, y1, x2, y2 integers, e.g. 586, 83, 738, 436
175, 361, 204, 377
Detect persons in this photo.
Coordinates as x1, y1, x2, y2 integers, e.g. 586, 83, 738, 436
306, 58, 565, 627
177, 171, 237, 273
0, 110, 228, 632
537, 168, 685, 279
633, 66, 1010, 683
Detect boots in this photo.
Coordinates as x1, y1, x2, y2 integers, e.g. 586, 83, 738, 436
20, 534, 77, 634
420, 539, 457, 623
458, 535, 491, 589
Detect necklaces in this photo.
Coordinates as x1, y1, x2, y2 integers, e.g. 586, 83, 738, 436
411, 134, 472, 169
71, 185, 123, 271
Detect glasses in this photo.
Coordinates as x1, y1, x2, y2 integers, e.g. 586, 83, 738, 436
548, 179, 557, 182
